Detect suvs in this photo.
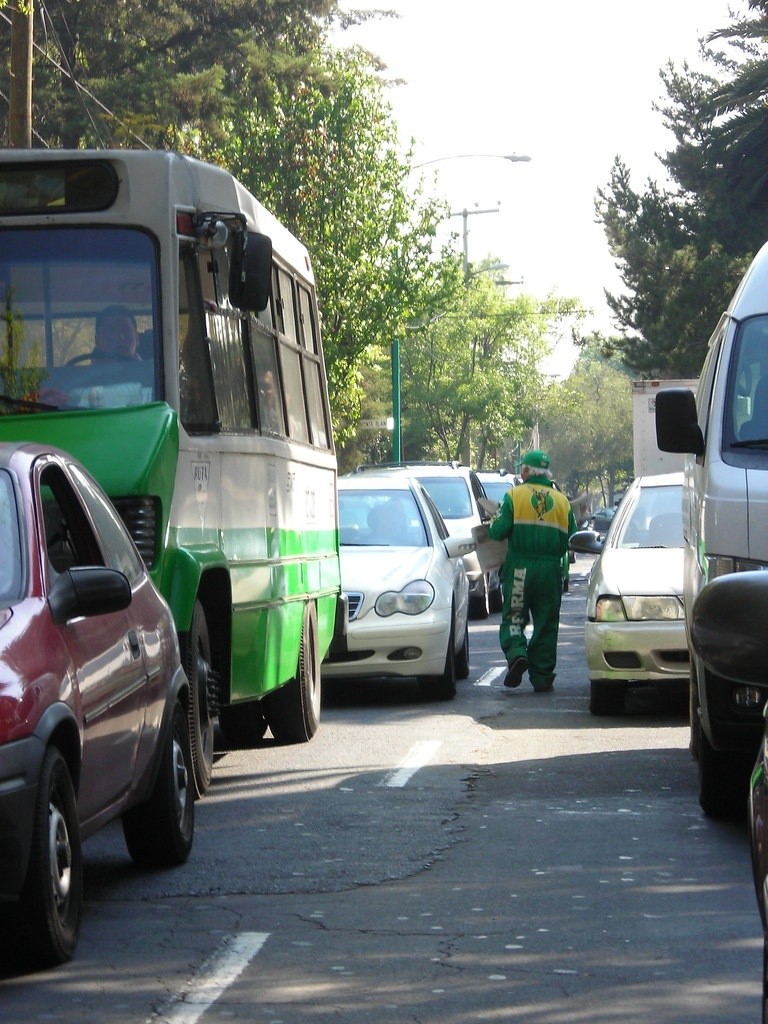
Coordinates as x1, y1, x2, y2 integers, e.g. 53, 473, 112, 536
0, 440, 196, 973
341, 461, 521, 619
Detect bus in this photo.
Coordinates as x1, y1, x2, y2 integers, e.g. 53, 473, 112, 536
0, 151, 348, 800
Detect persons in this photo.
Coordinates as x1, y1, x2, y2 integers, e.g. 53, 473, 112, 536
91, 306, 143, 363
488, 452, 579, 691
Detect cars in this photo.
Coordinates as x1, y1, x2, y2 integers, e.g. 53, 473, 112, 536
568, 472, 685, 717
320, 476, 477, 699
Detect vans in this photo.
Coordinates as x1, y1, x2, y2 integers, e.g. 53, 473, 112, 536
654, 238, 768, 827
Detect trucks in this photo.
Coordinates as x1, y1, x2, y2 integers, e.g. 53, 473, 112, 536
632, 380, 699, 480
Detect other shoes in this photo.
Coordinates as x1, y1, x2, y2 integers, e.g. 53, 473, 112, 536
504, 657, 529, 689
534, 684, 554, 692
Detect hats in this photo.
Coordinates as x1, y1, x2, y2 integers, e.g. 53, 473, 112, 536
513, 450, 550, 470
